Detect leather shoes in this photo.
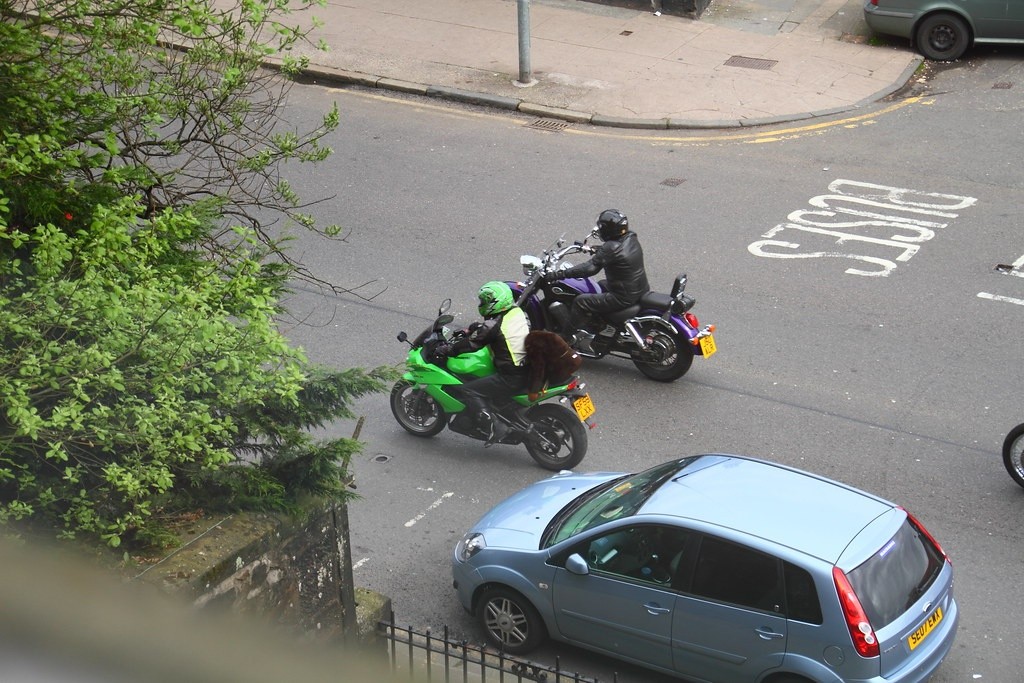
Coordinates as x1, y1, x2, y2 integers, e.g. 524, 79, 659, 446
484, 418, 512, 449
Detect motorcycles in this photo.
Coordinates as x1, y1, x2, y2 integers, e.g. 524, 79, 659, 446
391, 298, 596, 471
504, 226, 717, 383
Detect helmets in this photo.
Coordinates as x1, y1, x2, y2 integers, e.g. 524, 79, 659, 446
477, 280, 515, 316
596, 209, 629, 241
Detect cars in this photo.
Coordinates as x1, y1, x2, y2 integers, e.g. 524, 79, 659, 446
451, 452, 959, 683
865, 0, 1024, 62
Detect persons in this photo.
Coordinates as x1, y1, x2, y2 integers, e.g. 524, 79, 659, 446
432, 281, 530, 448
543, 209, 649, 343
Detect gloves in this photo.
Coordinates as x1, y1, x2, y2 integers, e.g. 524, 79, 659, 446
432, 345, 455, 359
469, 321, 484, 332
544, 269, 565, 283
590, 245, 603, 254
